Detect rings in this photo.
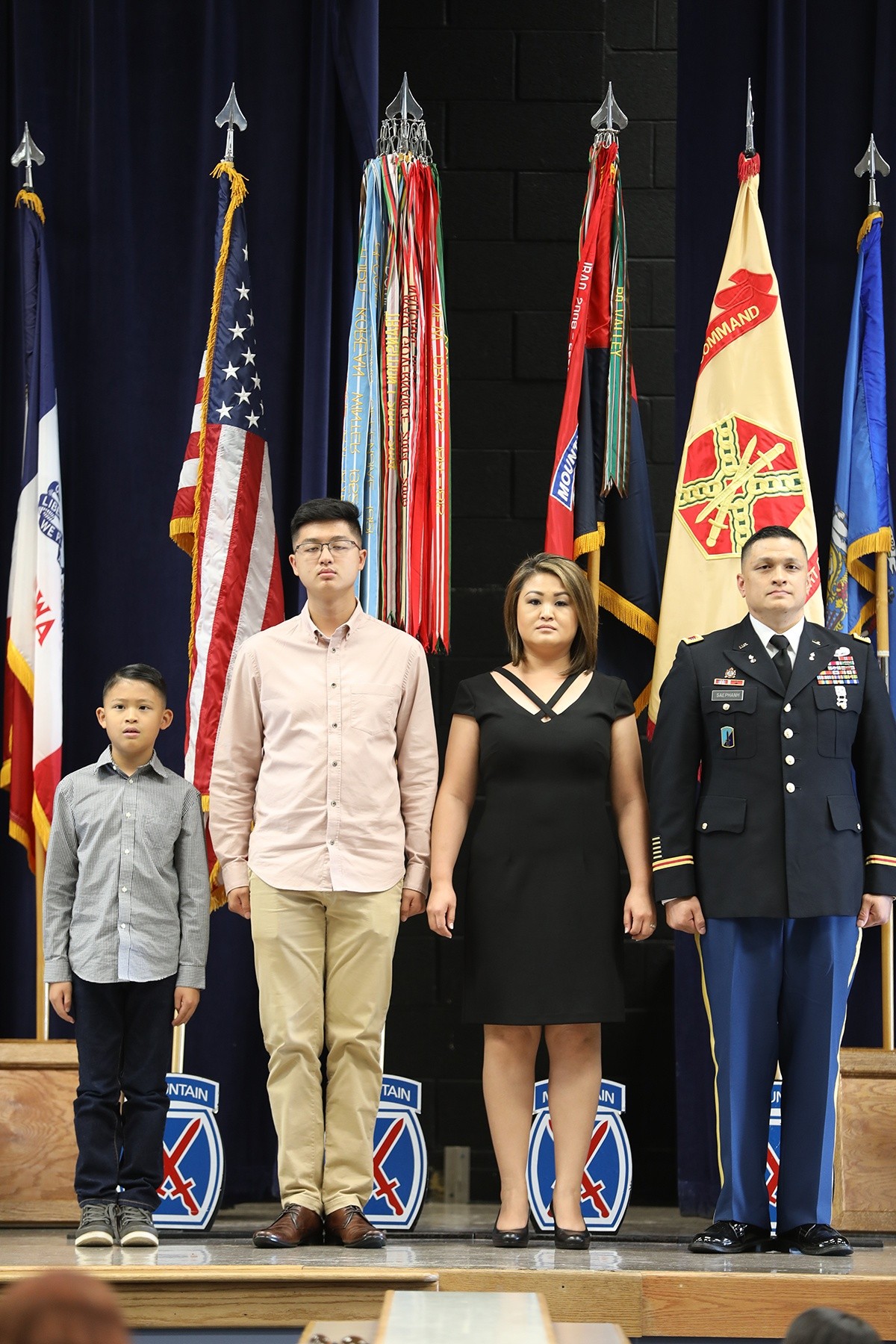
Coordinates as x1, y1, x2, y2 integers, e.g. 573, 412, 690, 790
649, 924, 657, 929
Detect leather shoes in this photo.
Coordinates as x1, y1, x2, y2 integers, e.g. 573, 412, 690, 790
688, 1220, 772, 1253
253, 1204, 322, 1248
776, 1222, 854, 1256
323, 1204, 387, 1247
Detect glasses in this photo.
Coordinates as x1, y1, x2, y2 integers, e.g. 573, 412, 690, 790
293, 539, 361, 558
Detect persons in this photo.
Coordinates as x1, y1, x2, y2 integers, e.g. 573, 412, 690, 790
43, 663, 211, 1247
0, 1273, 129, 1344
208, 498, 439, 1249
425, 552, 658, 1249
648, 526, 896, 1256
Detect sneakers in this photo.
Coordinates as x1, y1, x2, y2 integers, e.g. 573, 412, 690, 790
75, 1203, 117, 1246
116, 1202, 159, 1246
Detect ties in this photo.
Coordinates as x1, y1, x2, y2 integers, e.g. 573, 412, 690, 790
768, 635, 792, 690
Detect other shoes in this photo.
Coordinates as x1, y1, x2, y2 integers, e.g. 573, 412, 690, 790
491, 1201, 530, 1246
550, 1198, 591, 1249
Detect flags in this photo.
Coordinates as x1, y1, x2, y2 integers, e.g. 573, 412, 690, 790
0, 142, 896, 912
